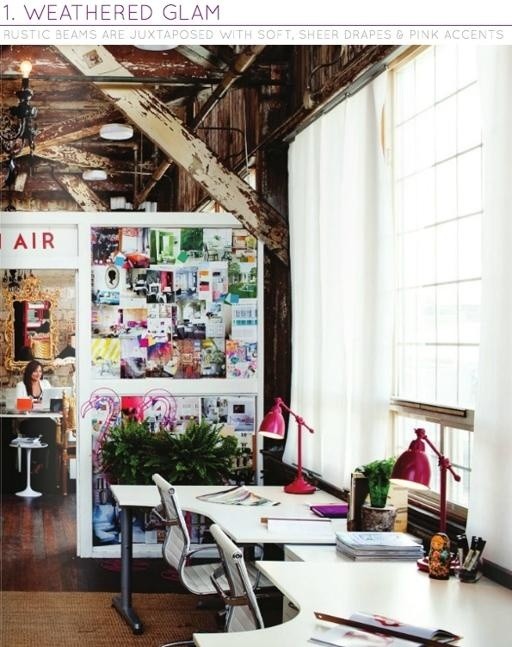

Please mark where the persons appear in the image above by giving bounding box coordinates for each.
[16,360,53,474]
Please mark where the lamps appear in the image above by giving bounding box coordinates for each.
[258,397,316,493]
[0,60,38,212]
[387,427,461,575]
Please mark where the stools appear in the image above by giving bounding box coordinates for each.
[10,442,49,497]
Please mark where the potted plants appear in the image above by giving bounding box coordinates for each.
[354,455,396,532]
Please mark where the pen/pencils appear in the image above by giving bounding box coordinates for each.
[456,534,486,580]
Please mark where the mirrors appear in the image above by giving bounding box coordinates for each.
[3,276,62,372]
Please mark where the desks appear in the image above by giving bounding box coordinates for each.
[1,411,63,488]
[108,484,512,645]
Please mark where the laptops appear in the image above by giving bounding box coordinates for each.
[34,386,72,411]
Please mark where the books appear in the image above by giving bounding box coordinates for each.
[309,505,348,518]
[311,608,461,647]
[334,530,426,562]
